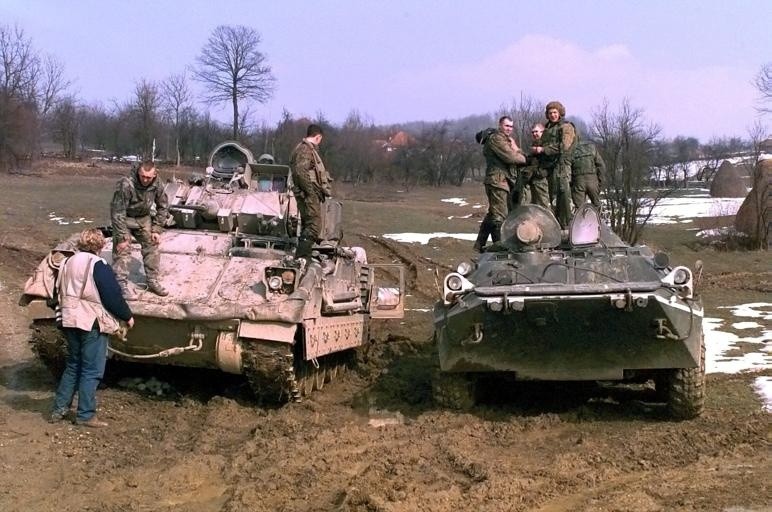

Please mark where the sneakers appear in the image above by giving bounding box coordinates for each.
[47,408,76,423]
[472,242,484,253]
[147,279,169,295]
[121,287,138,300]
[298,258,326,269]
[74,414,108,428]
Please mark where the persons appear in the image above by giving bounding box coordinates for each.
[569,140,607,212]
[110,160,169,300]
[529,101,577,226]
[472,116,527,252]
[52,227,135,428]
[288,123,332,268]
[522,123,550,210]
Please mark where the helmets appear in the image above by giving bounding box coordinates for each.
[476,128,498,144]
[546,102,565,119]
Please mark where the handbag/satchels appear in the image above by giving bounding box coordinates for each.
[312,161,334,196]
[55,304,62,330]
[540,161,556,177]
